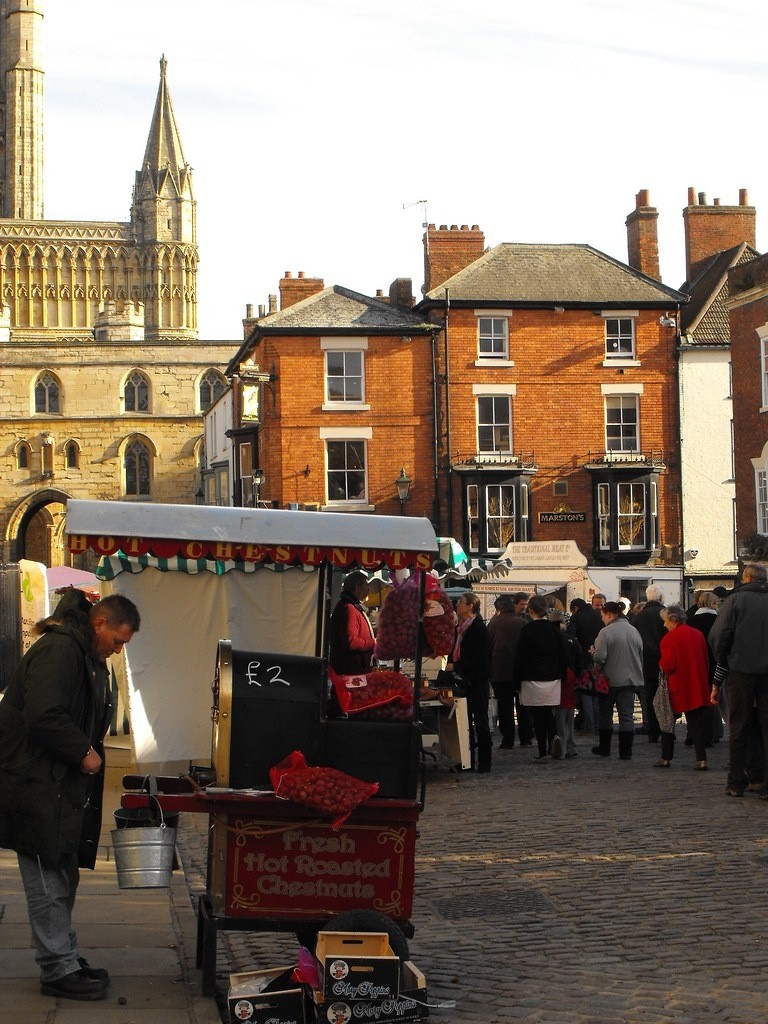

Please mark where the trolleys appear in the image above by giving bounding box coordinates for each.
[63,497,439,968]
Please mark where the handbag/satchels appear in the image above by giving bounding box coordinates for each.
[518,678,562,707]
[653,669,674,734]
[433,667,465,689]
[572,649,609,698]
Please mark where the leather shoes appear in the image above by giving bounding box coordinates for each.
[43,955,110,1001]
[693,760,706,771]
[653,758,670,767]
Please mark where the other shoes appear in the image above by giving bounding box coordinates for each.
[685,736,692,747]
[520,739,532,748]
[533,748,578,763]
[550,735,562,758]
[635,723,650,735]
[704,735,719,749]
[724,782,768,800]
[499,744,513,750]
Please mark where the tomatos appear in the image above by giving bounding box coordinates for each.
[278,587,456,814]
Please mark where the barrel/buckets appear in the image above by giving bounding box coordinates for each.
[113,773,182,871]
[110,795,176,889]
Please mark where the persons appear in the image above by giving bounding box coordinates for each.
[446,563,768,797]
[327,569,380,678]
[0,591,141,1000]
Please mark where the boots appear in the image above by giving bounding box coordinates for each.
[470,732,492,772]
[618,729,633,760]
[590,729,611,757]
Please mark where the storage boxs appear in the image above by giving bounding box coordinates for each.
[317,931,400,1001]
[229,966,307,1024]
[313,961,430,1024]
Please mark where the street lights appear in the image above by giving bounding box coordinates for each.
[394,469,412,516]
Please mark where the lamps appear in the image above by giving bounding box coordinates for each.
[395,468,411,503]
